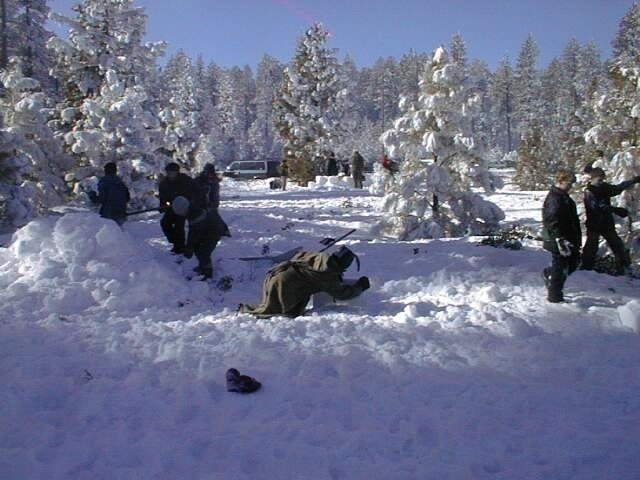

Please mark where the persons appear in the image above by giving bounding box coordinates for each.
[541,168,582,302]
[237,246,370,318]
[324,151,364,189]
[88,162,232,283]
[580,167,639,271]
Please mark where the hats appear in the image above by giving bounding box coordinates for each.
[166,163,179,171]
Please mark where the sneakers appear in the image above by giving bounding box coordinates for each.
[226,368,261,393]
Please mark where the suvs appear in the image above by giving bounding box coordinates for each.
[213,158,284,182]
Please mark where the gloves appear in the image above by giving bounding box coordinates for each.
[356,275,369,291]
[556,237,575,257]
